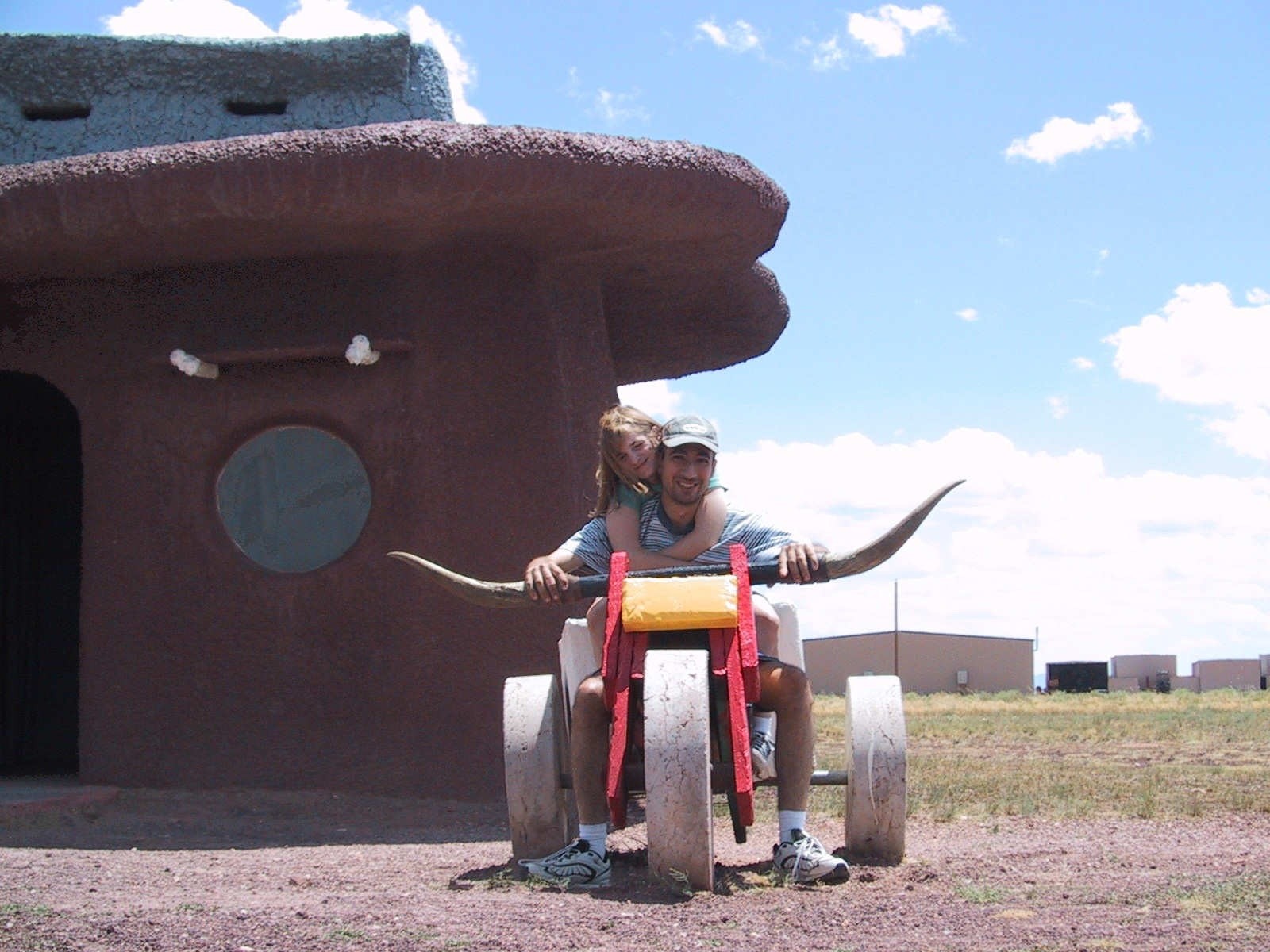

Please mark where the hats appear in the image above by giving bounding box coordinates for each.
[655,413,719,455]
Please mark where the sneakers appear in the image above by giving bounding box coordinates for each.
[516,838,613,889]
[749,729,777,766]
[772,829,851,884]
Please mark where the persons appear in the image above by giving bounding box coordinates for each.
[588,406,780,779]
[508,413,851,888]
